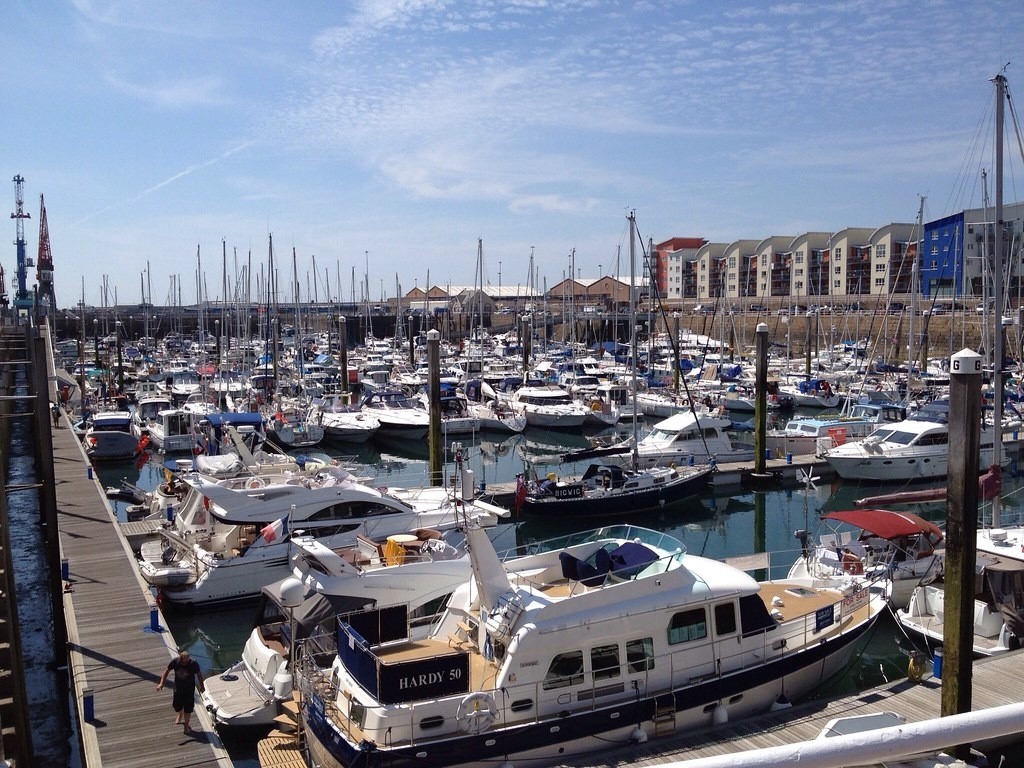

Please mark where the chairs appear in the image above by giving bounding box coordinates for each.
[560,541,660,589]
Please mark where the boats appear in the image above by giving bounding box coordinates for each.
[894,524,1024,655]
[199,530,473,727]
[139,411,515,605]
[282,526,916,768]
[787,508,947,608]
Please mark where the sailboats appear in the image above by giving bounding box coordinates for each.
[54,233,1024,525]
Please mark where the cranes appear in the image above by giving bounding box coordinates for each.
[8,172,36,309]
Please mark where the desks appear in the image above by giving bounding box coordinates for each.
[386,533,418,550]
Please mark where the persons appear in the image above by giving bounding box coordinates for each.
[51,403,62,428]
[156,651,206,733]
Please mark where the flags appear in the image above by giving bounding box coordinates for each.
[260,514,290,544]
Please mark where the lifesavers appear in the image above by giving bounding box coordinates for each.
[841,553,862,576]
[456,692,497,734]
[591,403,602,412]
[245,477,265,493]
[809,389,816,396]
[682,399,689,406]
[875,385,882,391]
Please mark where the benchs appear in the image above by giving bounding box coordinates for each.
[356,529,443,568]
